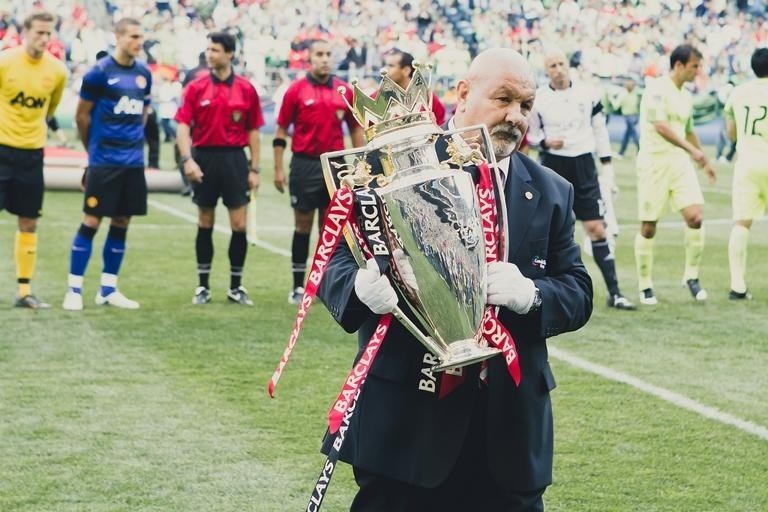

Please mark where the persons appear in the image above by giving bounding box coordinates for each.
[0,0,768,312]
[314,45,594,511]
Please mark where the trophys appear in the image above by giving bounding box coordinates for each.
[319,58,509,373]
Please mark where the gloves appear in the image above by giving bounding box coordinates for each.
[353,256,399,317]
[487,260,536,314]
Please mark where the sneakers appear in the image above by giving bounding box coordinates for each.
[681,276,707,301]
[288,286,305,306]
[13,292,51,309]
[94,288,140,311]
[728,287,754,301]
[226,284,255,307]
[191,285,212,305]
[62,288,83,311]
[639,286,658,306]
[603,292,638,309]
